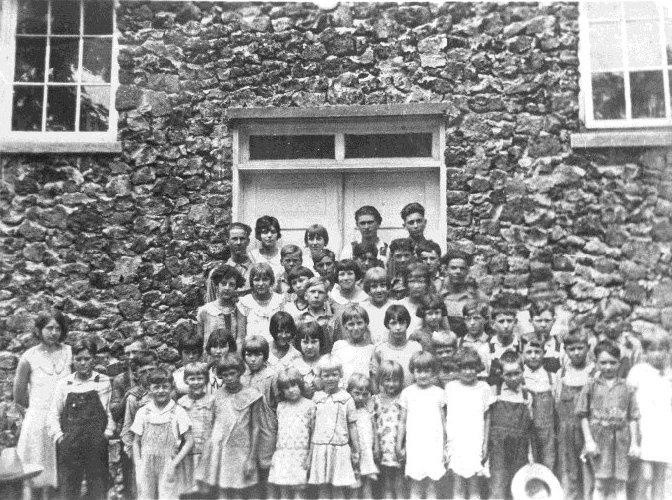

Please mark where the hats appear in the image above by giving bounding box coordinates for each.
[510,462,566,500]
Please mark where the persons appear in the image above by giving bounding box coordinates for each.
[14,309,216,500]
[479,268,671,500]
[199,202,495,500]
